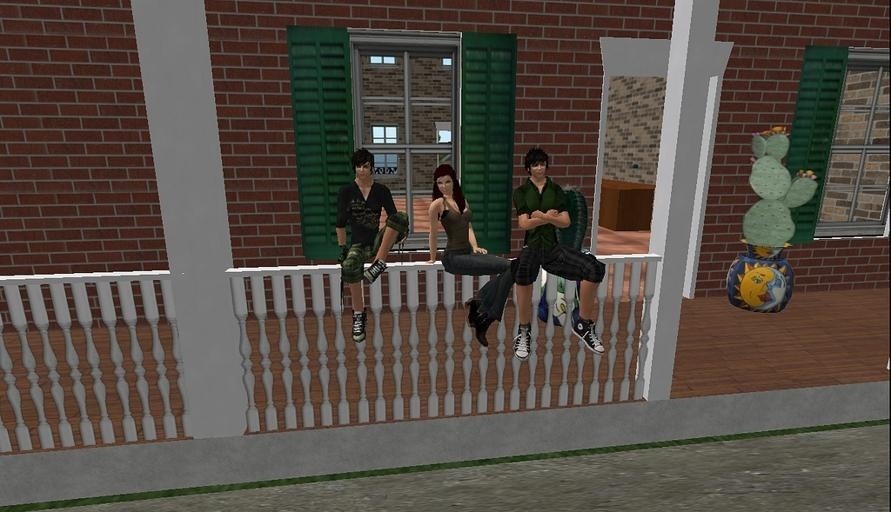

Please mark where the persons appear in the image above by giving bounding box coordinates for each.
[511,146,607,360]
[427,163,518,347]
[334,146,411,347]
